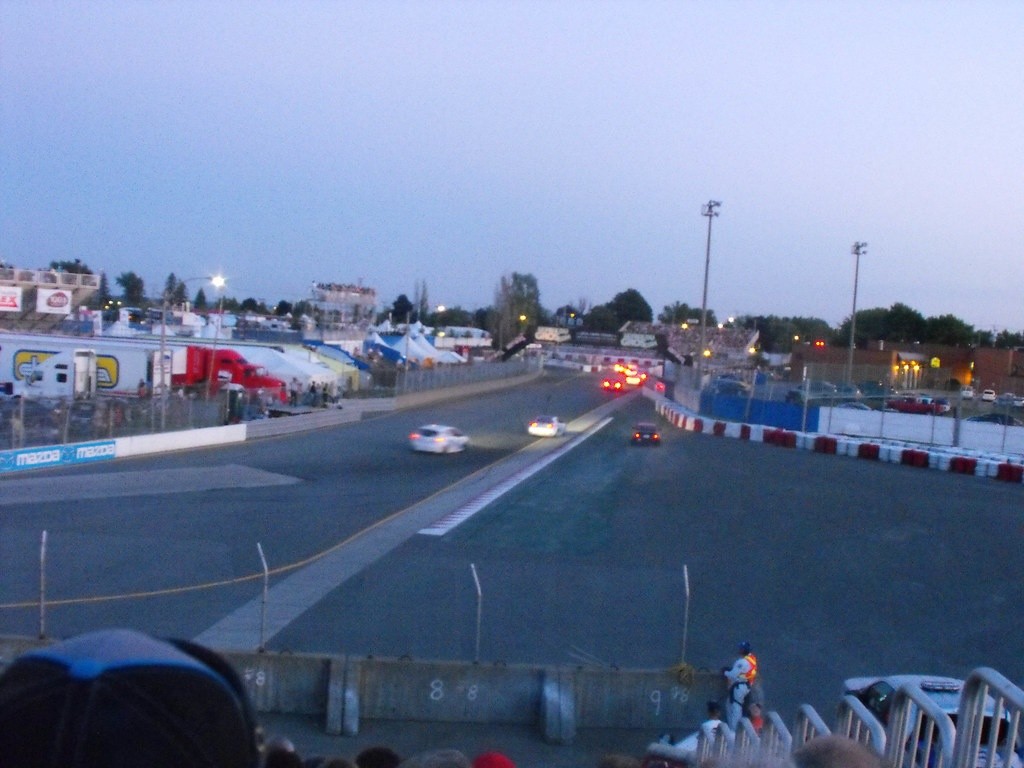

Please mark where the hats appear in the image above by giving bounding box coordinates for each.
[473,753,517,768]
[0,631,257,768]
[738,642,751,650]
[706,700,720,713]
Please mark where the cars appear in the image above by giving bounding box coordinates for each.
[798,378,1024,426]
[630,422,662,445]
[410,424,471,453]
[527,415,567,438]
[600,362,648,392]
[844,674,1024,768]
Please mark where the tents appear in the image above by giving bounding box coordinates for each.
[361,332,467,367]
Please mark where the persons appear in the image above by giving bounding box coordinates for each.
[700,639,763,737]
[290,377,329,409]
[0,629,892,767]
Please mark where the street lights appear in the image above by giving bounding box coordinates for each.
[698,199,721,360]
[160,275,224,399]
[846,240,868,381]
